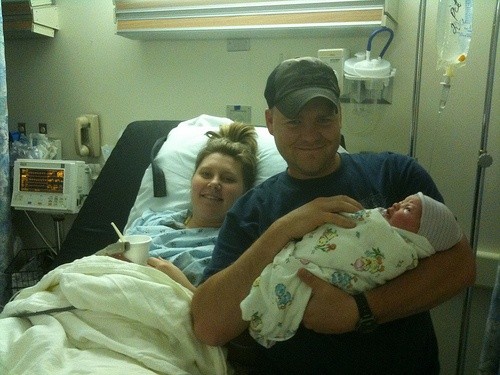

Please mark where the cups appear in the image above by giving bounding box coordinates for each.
[118,235,152,266]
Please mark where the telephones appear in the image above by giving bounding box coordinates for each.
[74,113,100,158]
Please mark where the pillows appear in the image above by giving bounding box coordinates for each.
[126,115,350,233]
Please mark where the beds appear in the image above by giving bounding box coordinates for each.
[57,120,304,375]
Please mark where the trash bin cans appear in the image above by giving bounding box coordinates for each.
[5,247,58,296]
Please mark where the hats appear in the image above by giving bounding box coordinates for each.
[415,192,465,253]
[263,56,341,120]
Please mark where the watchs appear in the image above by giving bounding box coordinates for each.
[353,293,377,334]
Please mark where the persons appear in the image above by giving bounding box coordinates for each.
[191,55,476,375]
[240,192,464,349]
[119,121,258,293]
